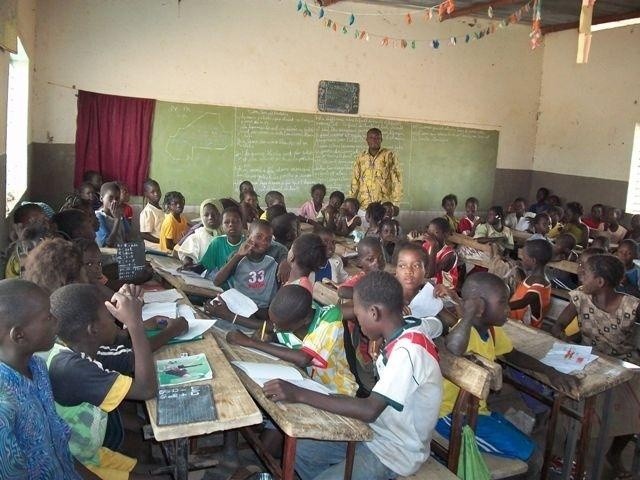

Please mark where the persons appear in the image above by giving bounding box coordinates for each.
[347,127,403,229]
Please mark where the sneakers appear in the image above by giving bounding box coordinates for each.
[569,459,590,480]
[548,455,564,475]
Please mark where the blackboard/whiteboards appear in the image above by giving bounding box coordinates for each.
[156,384,218,426]
[118,241,147,282]
[318,80,359,114]
[149,99,500,214]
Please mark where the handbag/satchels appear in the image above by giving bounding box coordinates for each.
[116,239,154,287]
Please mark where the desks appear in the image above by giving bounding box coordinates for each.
[496,320,639,480]
[136,252,374,479]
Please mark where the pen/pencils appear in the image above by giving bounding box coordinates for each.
[176,303,179,318]
[261,320,266,342]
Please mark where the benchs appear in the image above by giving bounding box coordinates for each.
[544,259,580,300]
[512,231,533,259]
[450,232,504,270]
[314,280,530,479]
